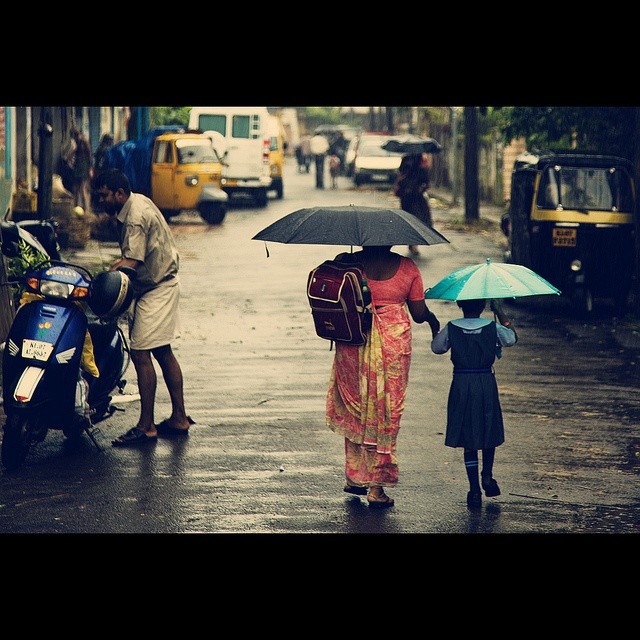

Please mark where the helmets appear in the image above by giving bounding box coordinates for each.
[87,268,134,320]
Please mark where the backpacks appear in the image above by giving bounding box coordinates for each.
[306,252,374,352]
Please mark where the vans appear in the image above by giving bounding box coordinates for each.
[354,136,402,183]
[188,107,272,206]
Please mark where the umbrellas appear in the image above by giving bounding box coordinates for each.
[379,132,446,168]
[426,258,563,324]
[251,201,451,258]
[314,125,339,133]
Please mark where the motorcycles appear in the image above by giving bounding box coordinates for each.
[2,220,130,467]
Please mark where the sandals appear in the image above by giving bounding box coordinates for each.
[343,485,368,496]
[368,493,395,508]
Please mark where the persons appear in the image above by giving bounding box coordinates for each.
[292,133,311,174]
[328,130,350,189]
[69,127,95,212]
[430,294,517,507]
[310,129,329,190]
[59,135,77,191]
[324,230,439,509]
[329,151,342,186]
[398,153,434,259]
[95,166,190,446]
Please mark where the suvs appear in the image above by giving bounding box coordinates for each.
[268,117,283,198]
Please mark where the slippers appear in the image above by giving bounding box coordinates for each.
[155,421,189,437]
[110,427,157,447]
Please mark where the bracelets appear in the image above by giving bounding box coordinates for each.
[502,321,511,327]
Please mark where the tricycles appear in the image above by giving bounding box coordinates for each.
[102,133,228,224]
[511,150,637,315]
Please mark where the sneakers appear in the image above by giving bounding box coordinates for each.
[467,492,482,508]
[482,477,501,497]
